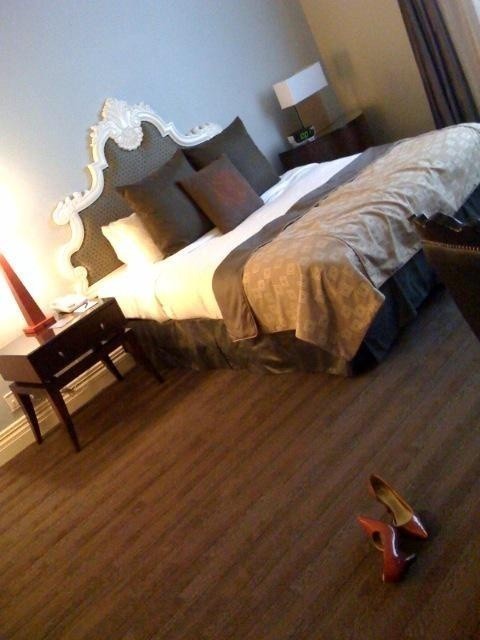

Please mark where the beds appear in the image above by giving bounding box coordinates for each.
[47,91,480,384]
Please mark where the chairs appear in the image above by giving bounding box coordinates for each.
[404,203,480,341]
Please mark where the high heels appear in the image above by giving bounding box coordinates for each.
[357,515,419,583]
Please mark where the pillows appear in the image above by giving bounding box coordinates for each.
[179,113,285,196]
[111,146,217,261]
[173,150,267,237]
[94,207,166,273]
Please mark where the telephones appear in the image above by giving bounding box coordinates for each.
[52,295,88,314]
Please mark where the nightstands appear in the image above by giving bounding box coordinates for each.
[0,292,165,455]
[274,106,376,174]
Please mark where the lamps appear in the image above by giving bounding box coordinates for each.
[268,57,338,144]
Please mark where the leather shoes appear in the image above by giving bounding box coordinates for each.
[368,474,429,539]
